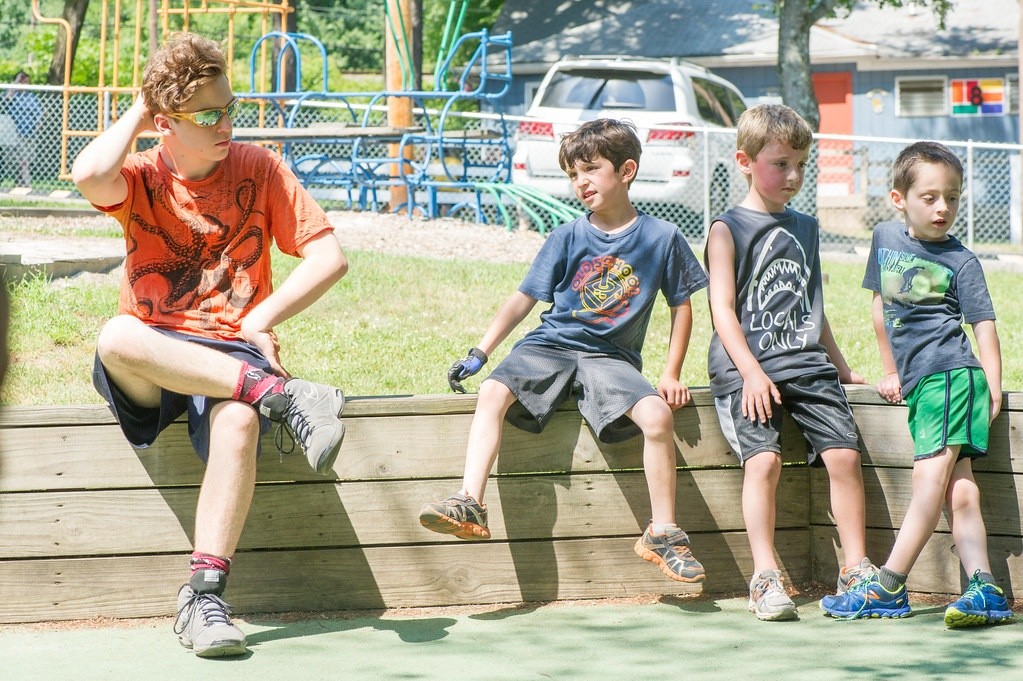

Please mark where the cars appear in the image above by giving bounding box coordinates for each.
[509,54,751,233]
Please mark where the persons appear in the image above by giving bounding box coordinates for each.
[818,143,1015,627]
[74,32,348,657]
[704,104,880,620]
[8,72,40,185]
[421,119,708,583]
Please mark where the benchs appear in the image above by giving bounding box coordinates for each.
[0,384,813,626]
[810,384,1023,600]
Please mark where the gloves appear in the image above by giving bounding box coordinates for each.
[447,347,489,394]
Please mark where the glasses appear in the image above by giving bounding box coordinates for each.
[166,95,241,128]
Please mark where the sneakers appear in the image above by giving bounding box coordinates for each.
[835,556,881,596]
[748,572,799,621]
[818,571,913,621]
[943,568,1015,629]
[418,489,491,541]
[173,568,247,656]
[634,518,707,583]
[258,376,346,477]
